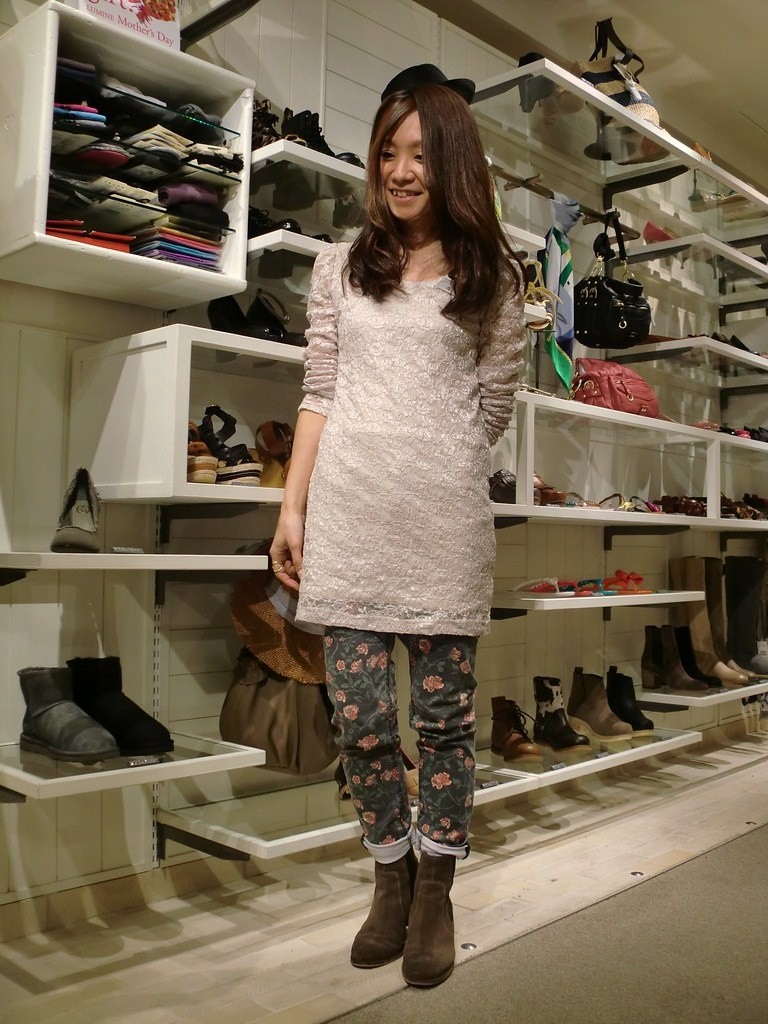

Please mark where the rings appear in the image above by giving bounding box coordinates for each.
[272,561,284,573]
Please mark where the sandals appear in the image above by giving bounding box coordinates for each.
[688,181,768,221]
[196,405,264,487]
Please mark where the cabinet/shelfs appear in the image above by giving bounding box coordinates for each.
[0,0,768,863]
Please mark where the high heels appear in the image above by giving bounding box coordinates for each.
[187,404,217,484]
[50,466,107,553]
[248,204,332,279]
[642,220,768,291]
[246,289,308,347]
[252,98,371,228]
[206,296,280,367]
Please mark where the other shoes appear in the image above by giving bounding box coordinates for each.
[538,84,585,124]
[517,53,555,113]
[490,468,768,530]
[686,330,768,378]
[685,421,768,442]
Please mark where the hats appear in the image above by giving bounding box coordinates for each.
[381,63,476,110]
[227,538,328,685]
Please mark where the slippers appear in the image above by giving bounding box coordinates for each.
[507,568,654,599]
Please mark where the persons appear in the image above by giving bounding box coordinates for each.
[270,62,524,988]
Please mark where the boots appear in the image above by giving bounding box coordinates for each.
[669,555,761,685]
[14,666,119,764]
[723,555,768,678]
[66,656,177,754]
[490,623,723,764]
[349,844,456,987]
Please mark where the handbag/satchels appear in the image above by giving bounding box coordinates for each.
[569,18,674,162]
[219,650,342,776]
[571,356,660,418]
[573,211,653,350]
[248,421,295,488]
